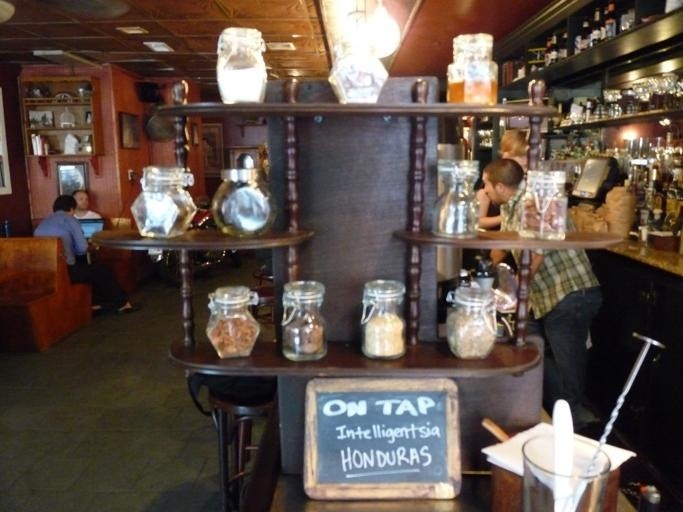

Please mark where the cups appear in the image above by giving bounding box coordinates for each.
[520,434,611,512]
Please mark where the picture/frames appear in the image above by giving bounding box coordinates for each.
[28,110,55,130]
[197,121,225,177]
[55,161,89,204]
[0,88,14,197]
[119,112,141,149]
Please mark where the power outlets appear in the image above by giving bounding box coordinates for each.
[128,169,135,182]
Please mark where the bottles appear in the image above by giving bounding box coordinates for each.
[205,284,261,360]
[278,279,331,363]
[518,167,569,244]
[209,168,282,239]
[215,26,269,105]
[430,159,481,242]
[443,283,501,361]
[130,163,199,240]
[329,24,390,105]
[446,31,499,107]
[358,279,408,362]
[543,0,682,67]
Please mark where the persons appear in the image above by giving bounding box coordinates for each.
[479,158,604,418]
[473,128,531,231]
[71,189,106,242]
[33,194,143,317]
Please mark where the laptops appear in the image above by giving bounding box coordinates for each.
[77,218,106,241]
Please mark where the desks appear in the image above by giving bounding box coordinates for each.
[239,397,640,512]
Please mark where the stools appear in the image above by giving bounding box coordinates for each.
[207,380,278,510]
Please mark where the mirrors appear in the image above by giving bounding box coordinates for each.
[227,144,262,169]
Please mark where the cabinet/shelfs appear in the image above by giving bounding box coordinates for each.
[164,76,544,379]
[464,2,681,245]
[591,250,683,478]
[19,76,98,160]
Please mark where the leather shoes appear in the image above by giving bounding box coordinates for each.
[94,305,113,314]
[120,303,140,313]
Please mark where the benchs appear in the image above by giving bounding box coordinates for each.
[0,235,92,353]
[27,217,133,294]
